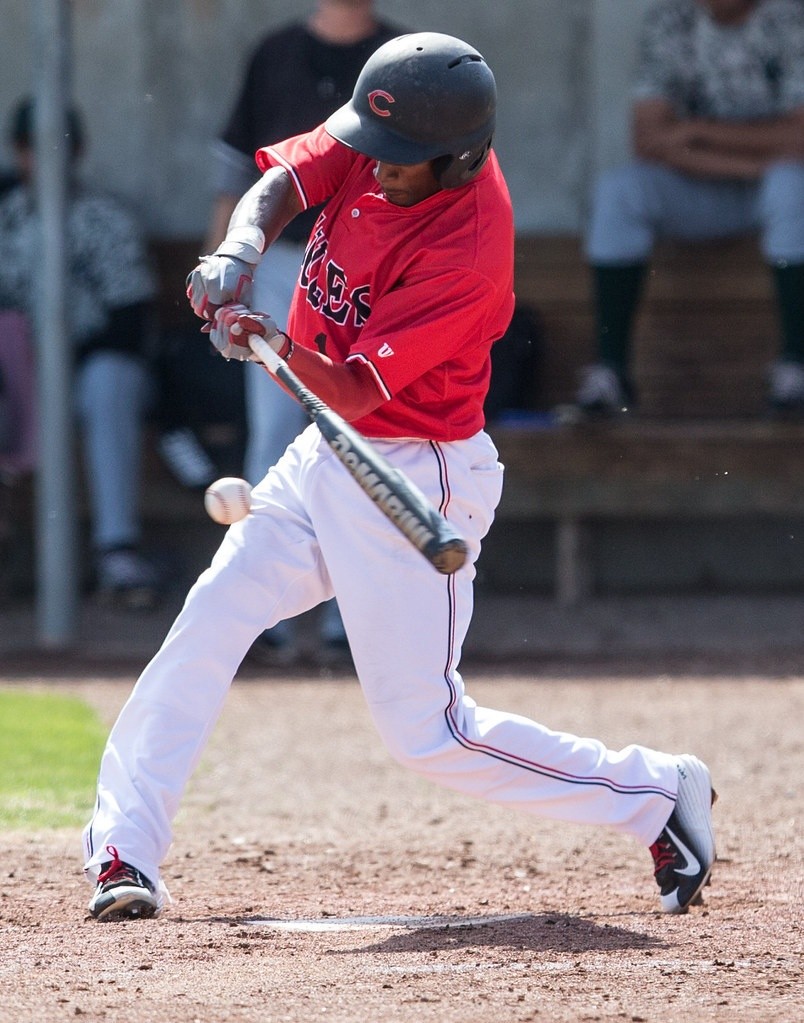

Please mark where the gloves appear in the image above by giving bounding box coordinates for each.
[186,241,261,333]
[211,303,285,362]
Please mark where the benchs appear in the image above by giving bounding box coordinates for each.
[0,411,804,608]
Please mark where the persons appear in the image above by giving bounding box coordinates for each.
[0,94,152,600]
[210,0,406,654]
[83,33,716,926]
[576,0,803,404]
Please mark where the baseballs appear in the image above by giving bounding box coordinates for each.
[204,476,252,525]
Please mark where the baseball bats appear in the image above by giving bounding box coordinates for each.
[248,333,470,577]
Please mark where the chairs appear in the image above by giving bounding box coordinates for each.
[206,194,783,419]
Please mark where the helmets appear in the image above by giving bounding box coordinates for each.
[324,31,497,188]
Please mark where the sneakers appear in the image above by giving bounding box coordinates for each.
[650,754,717,914]
[89,865,158,919]
